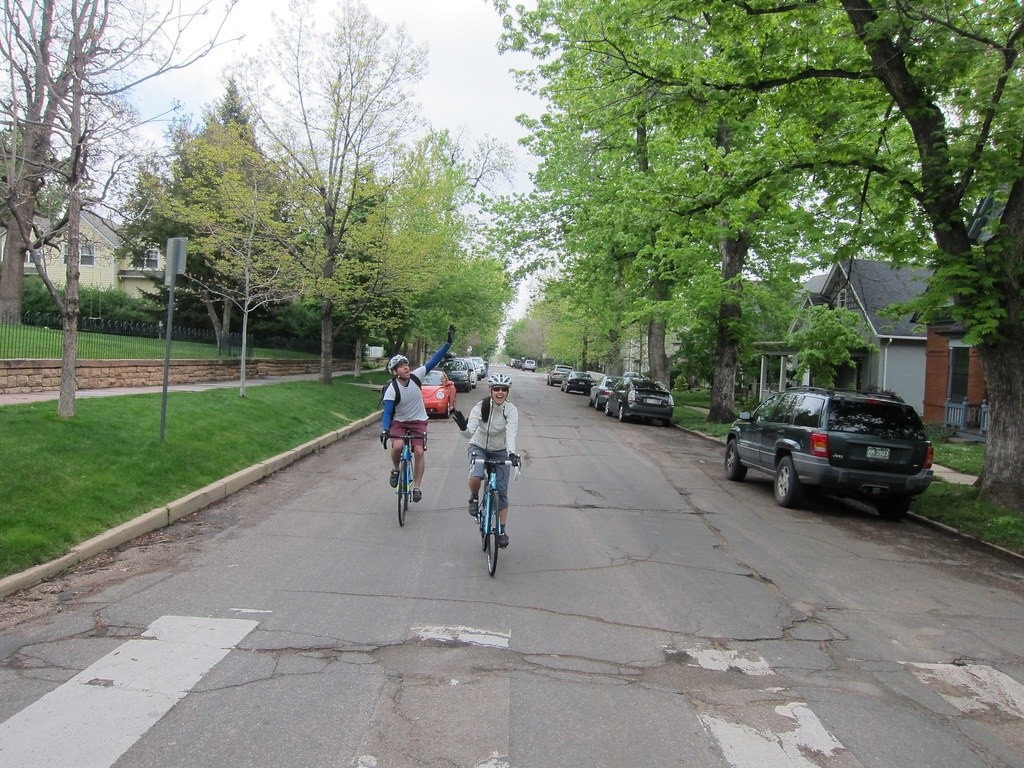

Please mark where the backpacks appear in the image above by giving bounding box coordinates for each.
[377,373,422,411]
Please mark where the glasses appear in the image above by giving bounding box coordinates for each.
[492,386,508,392]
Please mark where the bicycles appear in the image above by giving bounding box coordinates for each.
[380,431,427,527]
[468,453,522,576]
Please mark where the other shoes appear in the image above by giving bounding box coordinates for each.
[468,497,479,517]
[389,468,400,488]
[498,533,509,547]
[413,487,422,502]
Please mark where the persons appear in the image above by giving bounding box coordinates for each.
[379,323,457,502]
[159,320,163,339]
[453,373,519,547]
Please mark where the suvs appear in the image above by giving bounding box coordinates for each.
[522,360,537,372]
[546,365,573,386]
[724,386,935,521]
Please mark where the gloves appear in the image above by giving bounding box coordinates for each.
[509,452,519,467]
[380,431,390,443]
[452,409,469,431]
[447,324,457,343]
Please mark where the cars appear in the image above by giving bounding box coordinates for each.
[468,357,486,381]
[561,370,592,396]
[420,371,457,419]
[604,378,674,425]
[589,376,620,409]
[624,372,645,378]
[464,359,479,389]
[440,359,474,392]
[507,359,523,369]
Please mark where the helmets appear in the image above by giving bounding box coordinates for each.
[387,355,410,375]
[488,372,512,388]
